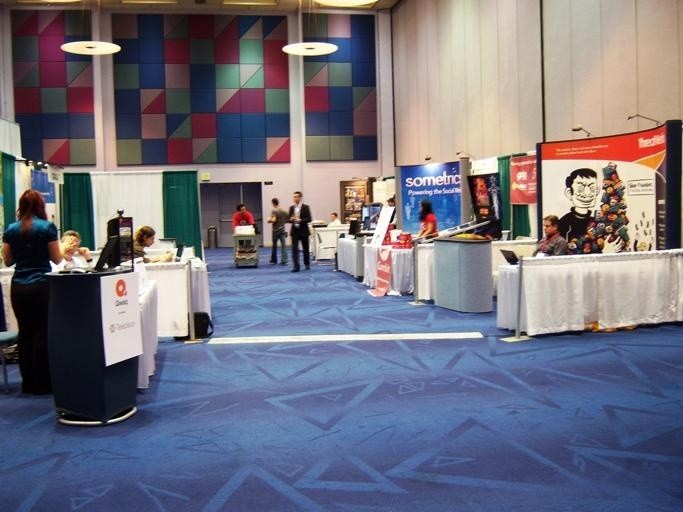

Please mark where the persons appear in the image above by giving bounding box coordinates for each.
[289,192,313,273]
[416,200,438,242]
[50,230,96,274]
[329,213,341,227]
[3,189,63,395]
[232,205,256,246]
[387,199,398,229]
[533,214,569,256]
[267,198,289,265]
[558,168,624,256]
[128,226,174,264]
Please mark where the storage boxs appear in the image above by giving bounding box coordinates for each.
[0,331,19,393]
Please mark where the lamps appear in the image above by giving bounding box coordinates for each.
[456,150,475,160]
[571,124,593,137]
[60,0,122,56]
[281,0,338,56]
[627,113,663,127]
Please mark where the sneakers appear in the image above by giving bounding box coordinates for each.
[279,262,288,266]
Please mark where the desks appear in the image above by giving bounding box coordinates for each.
[496,264,523,330]
[337,238,362,279]
[362,243,415,294]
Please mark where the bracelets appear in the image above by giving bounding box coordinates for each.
[88,257,94,264]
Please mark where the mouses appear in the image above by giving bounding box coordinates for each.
[72,268,85,273]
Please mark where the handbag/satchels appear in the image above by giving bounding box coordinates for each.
[174,312,214,342]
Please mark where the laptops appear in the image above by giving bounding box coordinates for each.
[501,250,520,265]
[176,245,183,258]
[76,235,118,273]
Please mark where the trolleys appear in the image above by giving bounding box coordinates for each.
[232,224,259,269]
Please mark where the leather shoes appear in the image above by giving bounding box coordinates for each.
[305,262,310,270]
[291,268,300,272]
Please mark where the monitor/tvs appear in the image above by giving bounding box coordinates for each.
[349,220,361,239]
[361,205,370,222]
